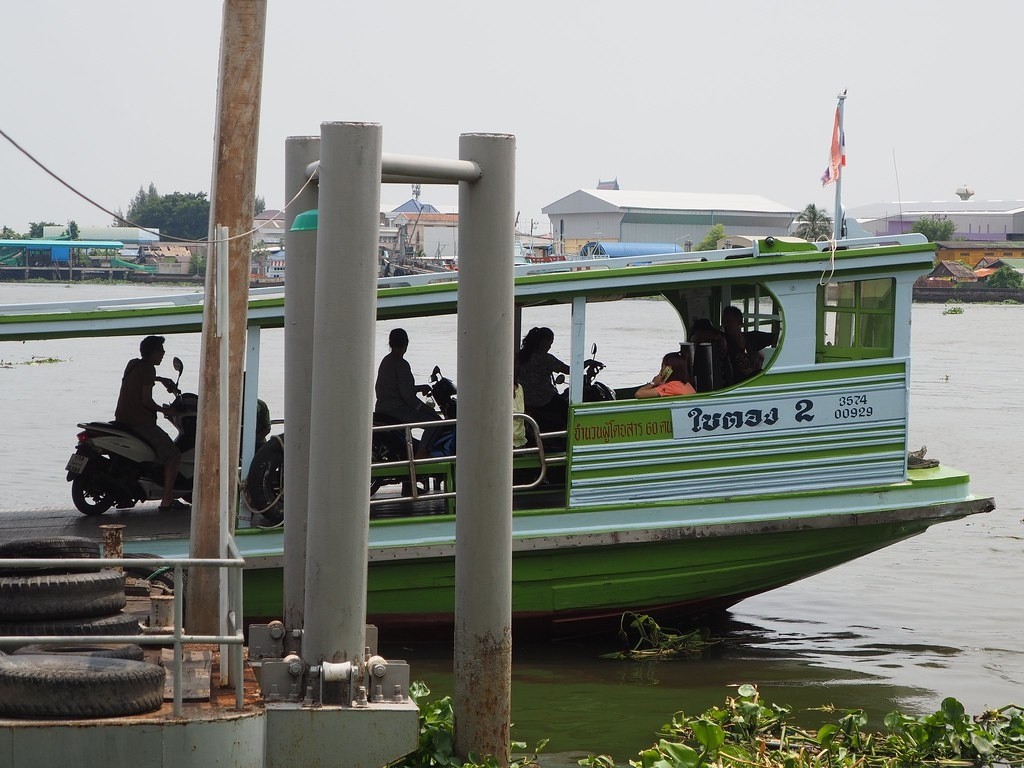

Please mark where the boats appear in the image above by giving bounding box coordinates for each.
[0,229,998,638]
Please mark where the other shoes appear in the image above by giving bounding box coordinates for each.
[401,483,427,496]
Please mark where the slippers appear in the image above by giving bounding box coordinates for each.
[158,500,189,511]
[116,501,135,508]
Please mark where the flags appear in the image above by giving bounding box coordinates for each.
[820,102,847,188]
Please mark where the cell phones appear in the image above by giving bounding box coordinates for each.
[660,366,673,382]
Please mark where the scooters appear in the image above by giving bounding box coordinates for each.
[525,342,618,452]
[65,356,199,517]
[371,365,458,496]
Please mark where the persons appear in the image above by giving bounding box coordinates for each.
[635,352,698,399]
[255,401,271,454]
[114,337,191,515]
[693,306,780,388]
[376,329,441,458]
[513,328,597,458]
[384,266,391,277]
